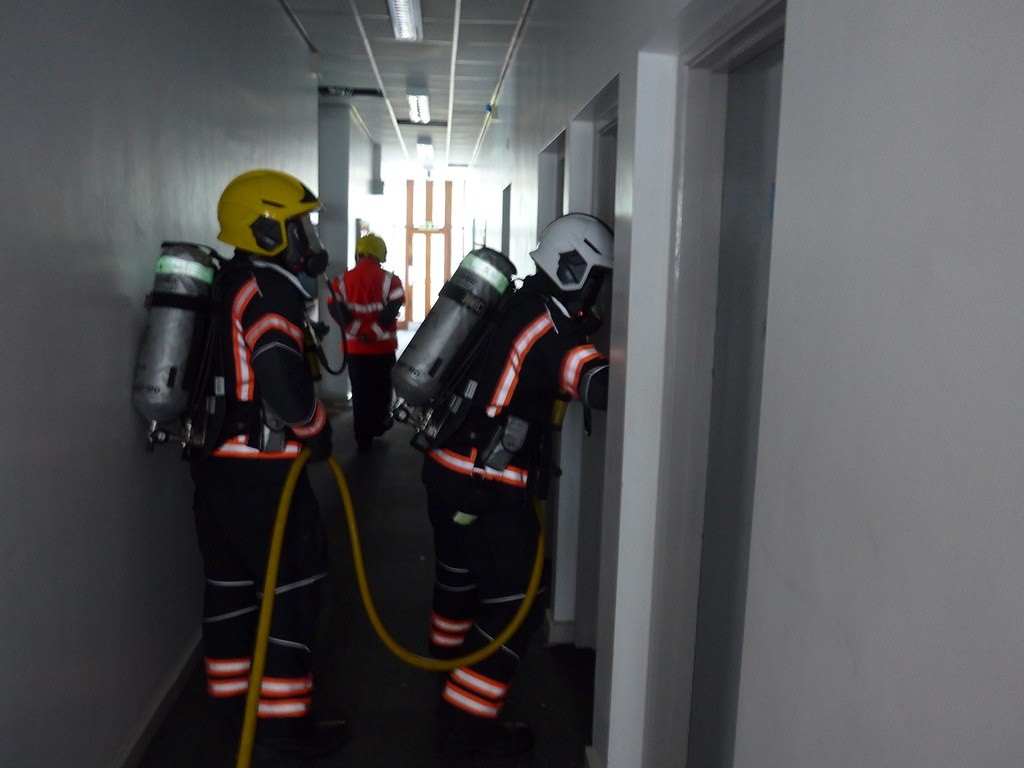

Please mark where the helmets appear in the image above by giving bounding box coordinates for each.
[530,212,612,291]
[355,235,387,263]
[217,171,320,255]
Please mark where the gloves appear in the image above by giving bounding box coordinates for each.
[299,420,333,464]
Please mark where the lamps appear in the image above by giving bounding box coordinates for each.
[388,0,423,42]
[406,89,430,125]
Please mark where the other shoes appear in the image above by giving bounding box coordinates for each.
[254,719,349,761]
[374,416,396,437]
[435,717,532,761]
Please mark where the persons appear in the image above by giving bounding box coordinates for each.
[423,211,614,760]
[197,170,352,761]
[327,233,405,445]
